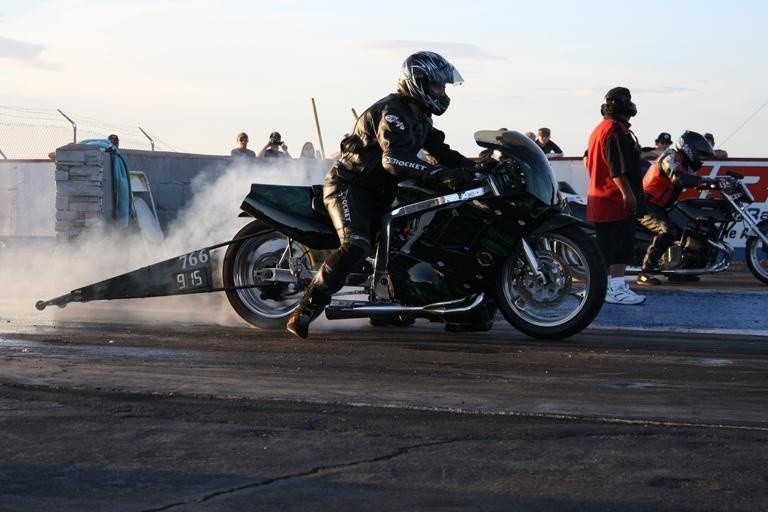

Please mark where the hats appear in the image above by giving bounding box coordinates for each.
[604,87,632,105]
[538,127,551,138]
[657,132,672,145]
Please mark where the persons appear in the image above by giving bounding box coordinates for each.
[640,132,673,161]
[583,87,647,306]
[230,132,256,157]
[107,134,120,148]
[633,130,726,286]
[535,128,563,159]
[703,133,728,159]
[287,50,500,340]
[299,141,315,159]
[257,131,292,158]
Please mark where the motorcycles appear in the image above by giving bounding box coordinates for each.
[221,129,609,341]
[554,167,767,283]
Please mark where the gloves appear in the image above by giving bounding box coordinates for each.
[715,175,738,194]
[425,163,474,193]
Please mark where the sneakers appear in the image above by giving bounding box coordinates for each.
[286,299,317,341]
[605,283,647,305]
[636,271,662,286]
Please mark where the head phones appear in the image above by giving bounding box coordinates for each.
[600,86,637,117]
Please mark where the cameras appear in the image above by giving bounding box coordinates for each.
[273,141,282,145]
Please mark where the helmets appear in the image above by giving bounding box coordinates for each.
[675,130,713,173]
[397,49,465,116]
